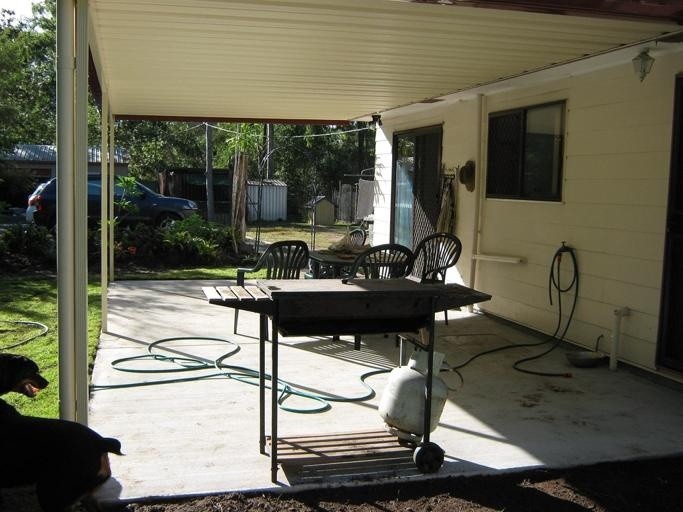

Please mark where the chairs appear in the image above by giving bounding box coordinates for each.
[330,242,417,354]
[229,239,311,338]
[306,228,368,279]
[404,231,463,327]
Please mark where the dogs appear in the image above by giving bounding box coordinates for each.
[0,353,126,511]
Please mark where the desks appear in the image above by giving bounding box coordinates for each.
[200,278,495,482]
[303,246,404,277]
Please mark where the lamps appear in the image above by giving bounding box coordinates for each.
[630,47,655,84]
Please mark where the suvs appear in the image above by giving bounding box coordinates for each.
[25,182,101,222]
[33,172,200,232]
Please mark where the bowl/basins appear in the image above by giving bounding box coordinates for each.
[563,350,604,368]
